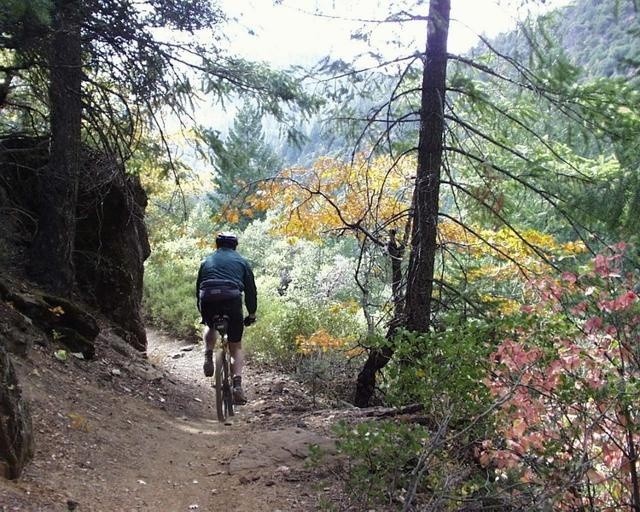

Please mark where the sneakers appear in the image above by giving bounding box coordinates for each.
[203,359,214,377]
[233,386,248,403]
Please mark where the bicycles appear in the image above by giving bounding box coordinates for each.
[198,315,257,421]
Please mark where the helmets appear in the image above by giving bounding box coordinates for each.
[215,231,238,248]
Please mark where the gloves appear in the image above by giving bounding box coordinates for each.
[244,314,256,327]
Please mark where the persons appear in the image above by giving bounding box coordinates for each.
[194,231,258,404]
